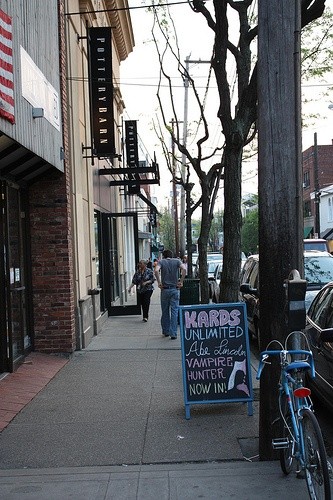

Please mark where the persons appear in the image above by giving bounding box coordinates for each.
[154,250,186,339]
[146,257,158,270]
[129,260,154,322]
[181,256,188,279]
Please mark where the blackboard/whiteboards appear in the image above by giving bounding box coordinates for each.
[179,302,255,404]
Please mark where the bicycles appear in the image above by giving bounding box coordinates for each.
[255,331,331,500]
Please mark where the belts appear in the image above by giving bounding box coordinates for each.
[163,286,179,289]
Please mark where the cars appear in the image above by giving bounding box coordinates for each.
[208,263,245,303]
[192,253,199,264]
[195,254,224,286]
[302,239,330,252]
[238,251,333,348]
[305,282,333,408]
